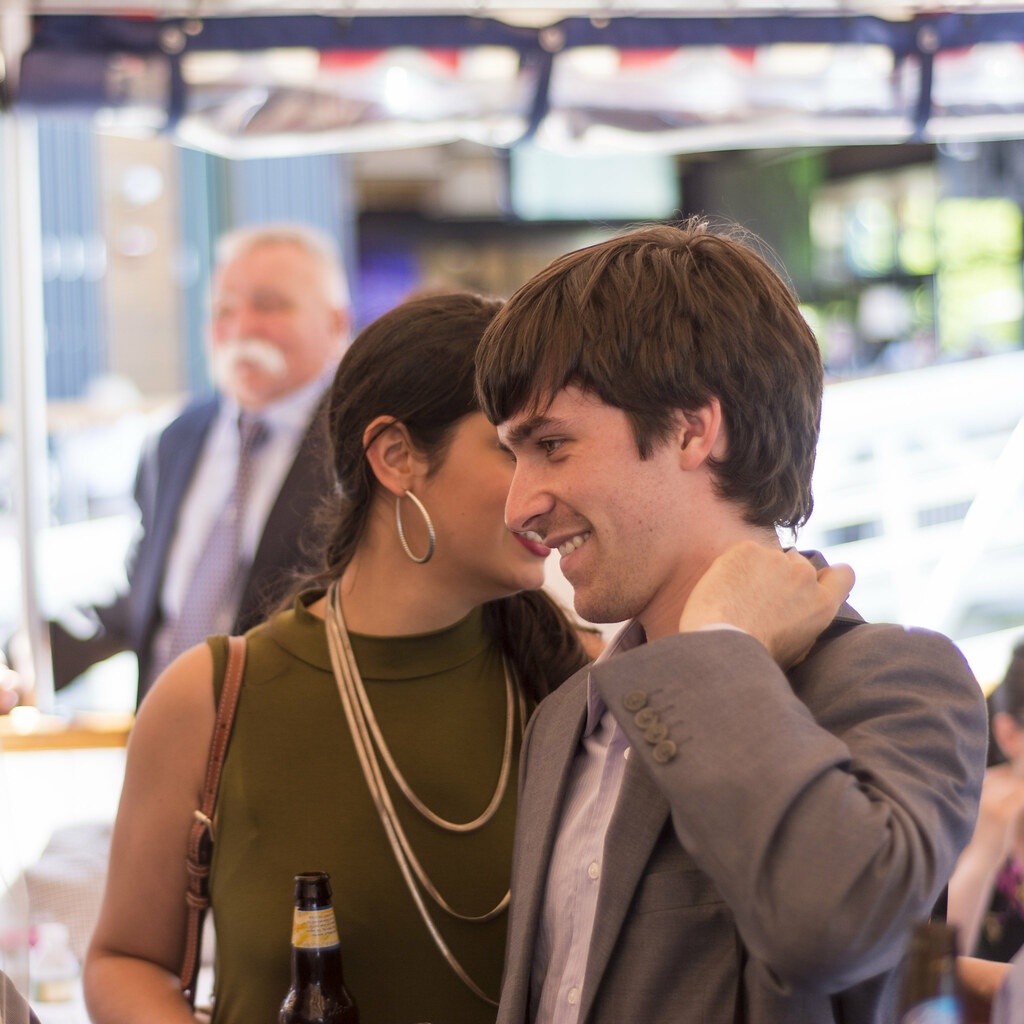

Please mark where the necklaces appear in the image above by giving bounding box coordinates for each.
[324,582,530,1007]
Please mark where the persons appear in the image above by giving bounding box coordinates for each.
[474,222,988,1024]
[0,229,351,719]
[947,639,1023,1024]
[83,293,610,1024]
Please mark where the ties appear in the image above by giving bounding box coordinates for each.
[166,413,274,670]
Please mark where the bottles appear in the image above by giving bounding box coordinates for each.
[892,920,971,1024]
[275,872,361,1024]
